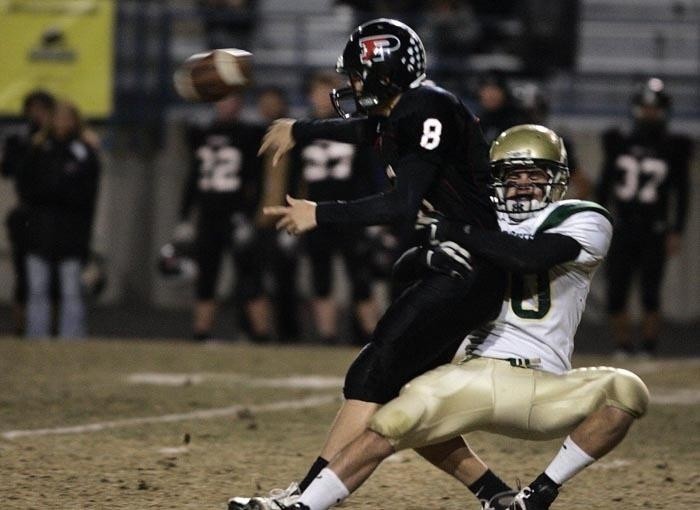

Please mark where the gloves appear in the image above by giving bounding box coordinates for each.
[412,210,476,285]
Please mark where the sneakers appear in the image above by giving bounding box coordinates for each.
[228,482,311,510]
[480,478,559,510]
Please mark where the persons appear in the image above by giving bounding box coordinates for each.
[245,72,588,343]
[591,78,691,361]
[228,16,519,510]
[251,125,649,510]
[0,89,104,339]
[174,95,276,342]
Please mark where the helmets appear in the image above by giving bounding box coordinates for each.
[335,17,426,101]
[628,77,673,116]
[489,123,571,201]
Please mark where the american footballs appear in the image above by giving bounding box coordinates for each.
[162,49,254,103]
